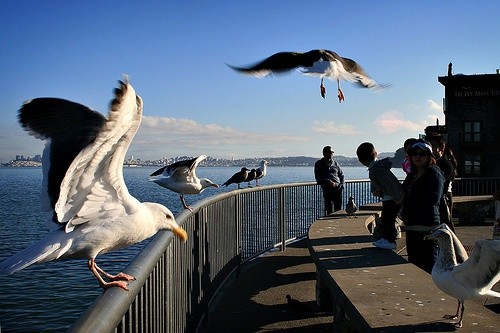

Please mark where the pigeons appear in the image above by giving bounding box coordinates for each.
[345,197,358,219]
[221,160,268,189]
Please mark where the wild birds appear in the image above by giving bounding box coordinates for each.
[148,154,219,215]
[0,73,188,292]
[423,222,499,328]
[223,48,393,104]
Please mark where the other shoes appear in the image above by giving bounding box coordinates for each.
[373,238,396,249]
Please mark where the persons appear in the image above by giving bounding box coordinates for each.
[401,132,457,274]
[314,146,345,216]
[357,142,405,249]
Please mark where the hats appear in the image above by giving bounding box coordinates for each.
[412,141,434,152]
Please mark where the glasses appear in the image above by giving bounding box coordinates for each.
[412,150,427,156]
